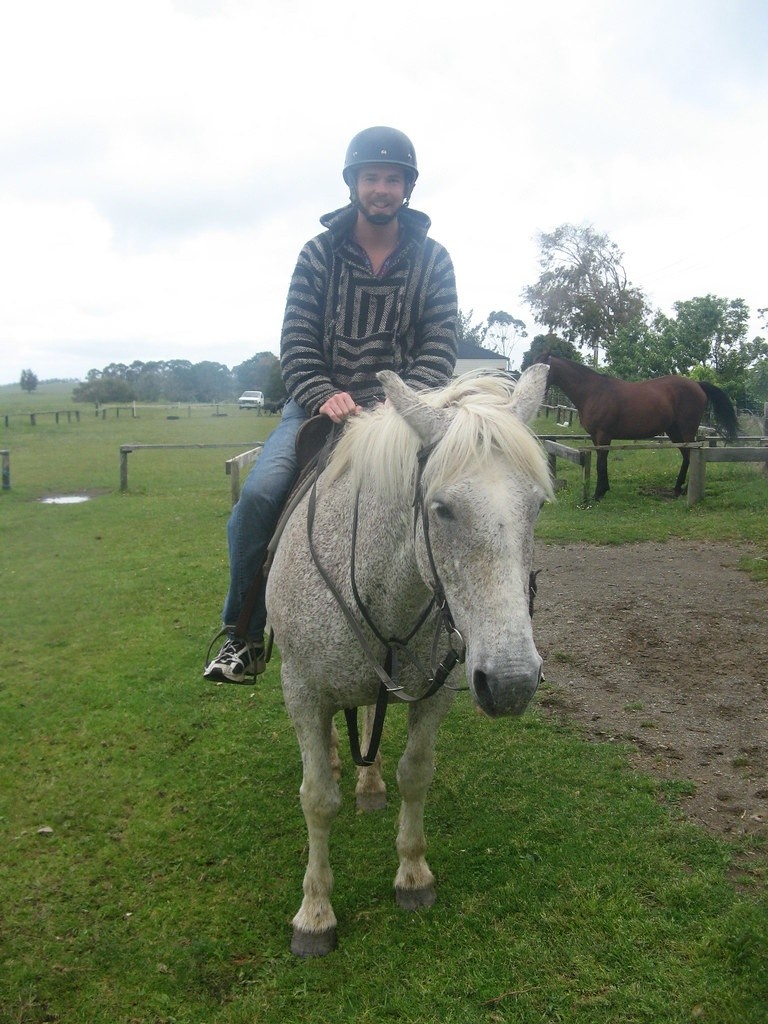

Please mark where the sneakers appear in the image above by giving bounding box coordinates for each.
[203,639,266,683]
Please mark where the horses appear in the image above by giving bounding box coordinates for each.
[265,364,557,957]
[533,350,747,503]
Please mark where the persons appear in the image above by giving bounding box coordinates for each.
[204,129,457,684]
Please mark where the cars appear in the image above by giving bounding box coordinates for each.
[238,390,265,408]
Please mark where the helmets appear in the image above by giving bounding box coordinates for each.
[343,126,418,175]
